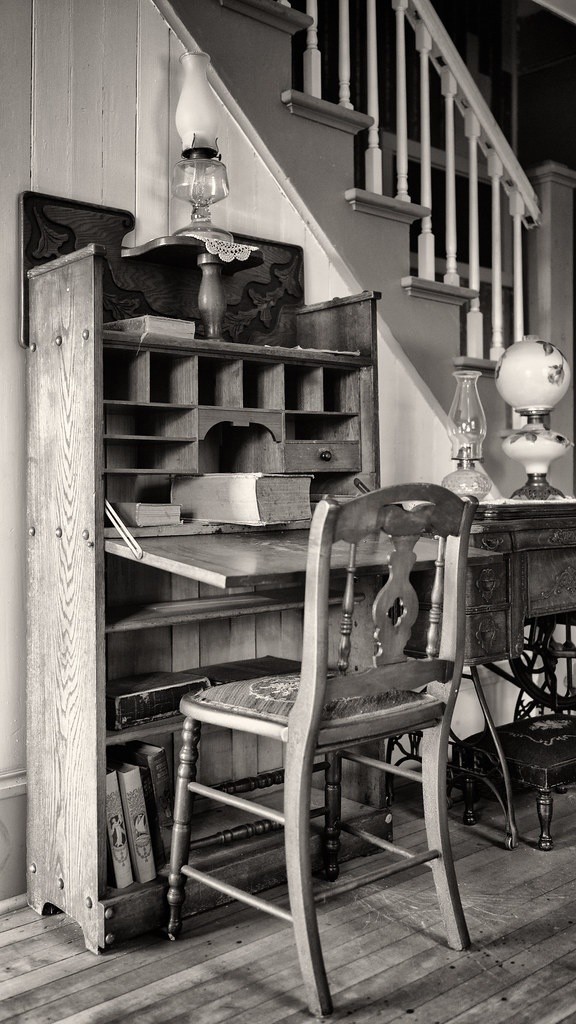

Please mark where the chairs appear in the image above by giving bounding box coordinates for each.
[166,482,479,1022]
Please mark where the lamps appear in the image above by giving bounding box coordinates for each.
[171,51,234,244]
[492,334,574,501]
[445,371,492,502]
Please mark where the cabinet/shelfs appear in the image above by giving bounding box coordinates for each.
[26,244,383,958]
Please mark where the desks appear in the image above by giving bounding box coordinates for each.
[393,501,576,851]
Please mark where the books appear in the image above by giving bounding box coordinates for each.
[103,315,196,339]
[106,740,174,889]
[107,671,211,731]
[109,501,183,527]
[170,472,315,526]
